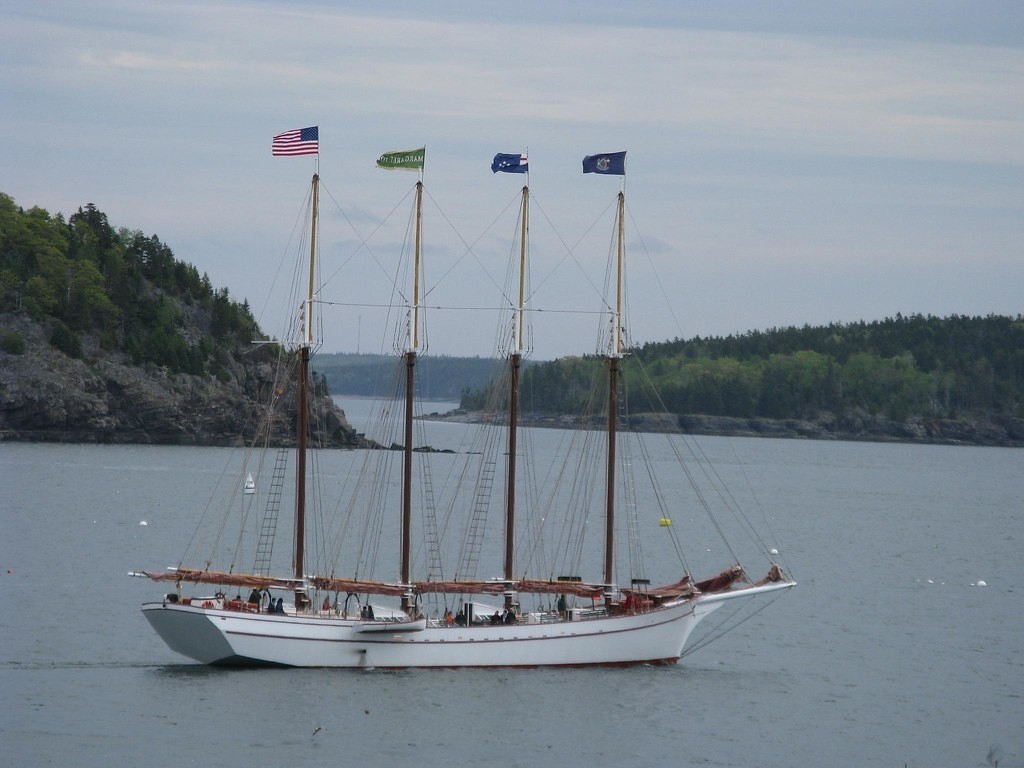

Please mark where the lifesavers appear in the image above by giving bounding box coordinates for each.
[202,601,215,608]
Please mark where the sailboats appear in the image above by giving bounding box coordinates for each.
[126,126,798,672]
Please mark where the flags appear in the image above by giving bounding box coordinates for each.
[375,148,425,172]
[272,126,318,156]
[583,151,627,175]
[491,152,529,174]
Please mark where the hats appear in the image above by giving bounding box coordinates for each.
[162,594,168,600]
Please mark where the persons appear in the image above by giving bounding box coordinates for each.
[268,598,287,615]
[446,611,454,627]
[558,594,567,619]
[455,610,466,627]
[232,596,246,610]
[361,605,374,620]
[163,594,179,604]
[491,609,516,626]
[249,589,261,612]
